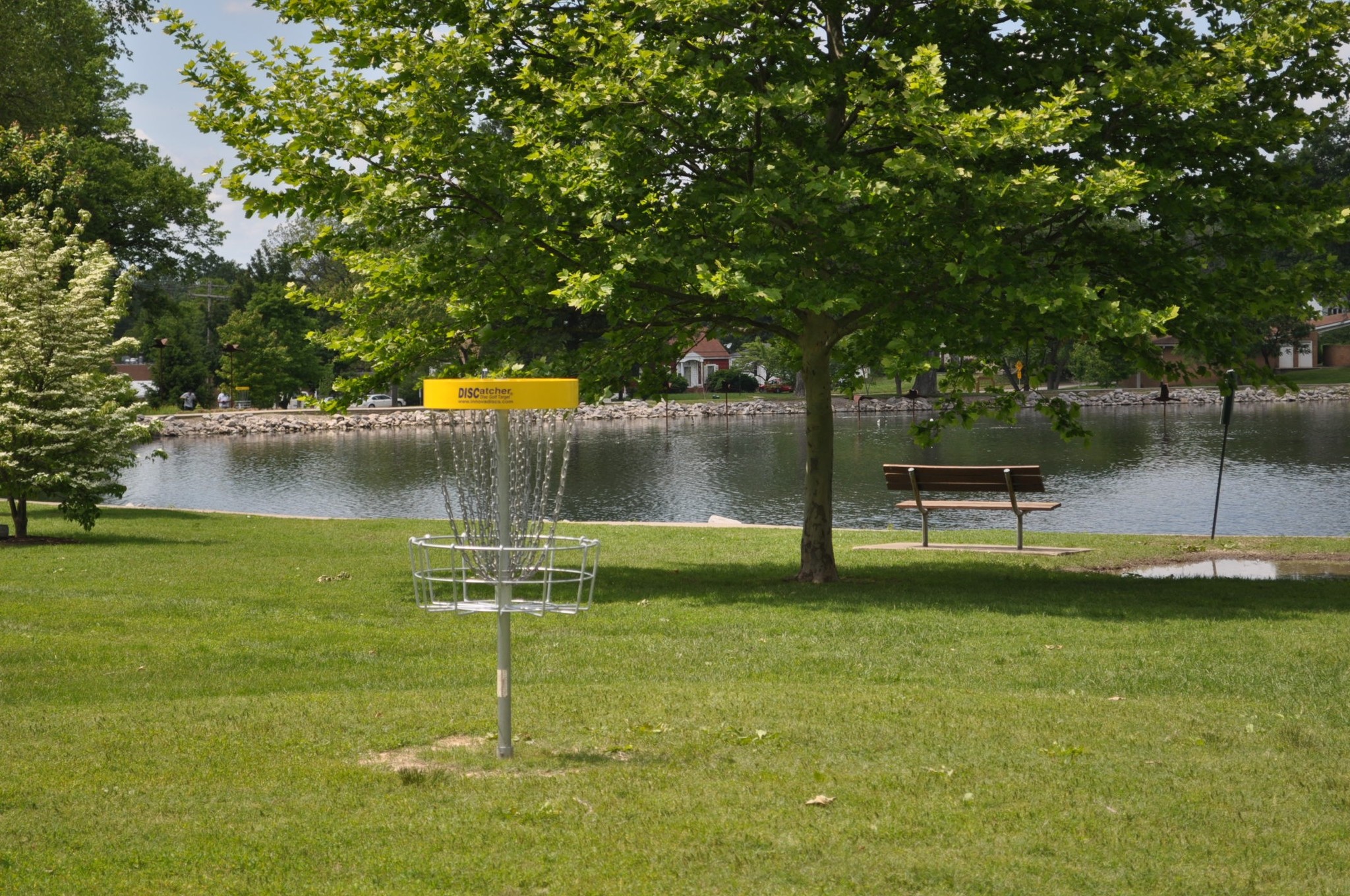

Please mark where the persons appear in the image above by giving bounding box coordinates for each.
[217,392,232,408]
[179,388,197,411]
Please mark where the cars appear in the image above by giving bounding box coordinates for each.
[758,379,793,393]
[287,392,313,409]
[356,393,407,408]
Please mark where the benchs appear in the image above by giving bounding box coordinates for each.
[883,464,1062,550]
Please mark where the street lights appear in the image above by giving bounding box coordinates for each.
[224,342,244,407]
[151,337,175,406]
